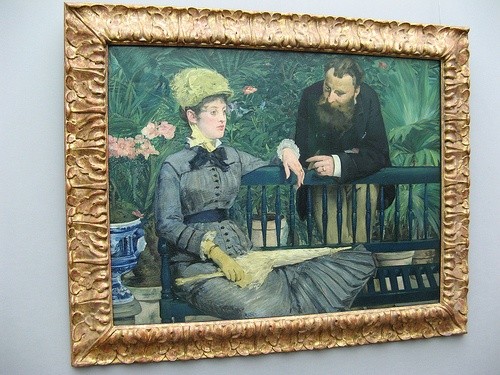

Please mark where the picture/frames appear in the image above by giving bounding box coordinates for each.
[61,1,472,370]
